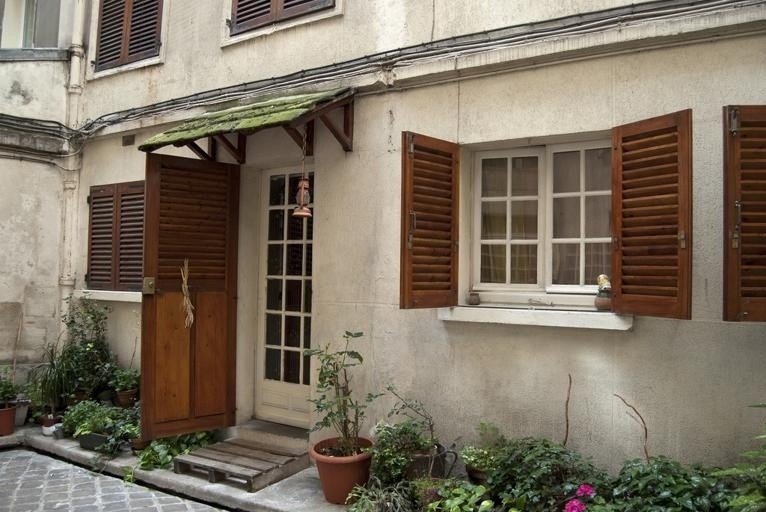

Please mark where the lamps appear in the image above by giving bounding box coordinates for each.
[290,129,315,221]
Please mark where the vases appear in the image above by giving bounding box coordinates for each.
[594,290,612,311]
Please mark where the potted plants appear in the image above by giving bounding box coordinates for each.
[377,382,461,487]
[461,418,504,486]
[1,294,160,455]
[301,329,378,505]
